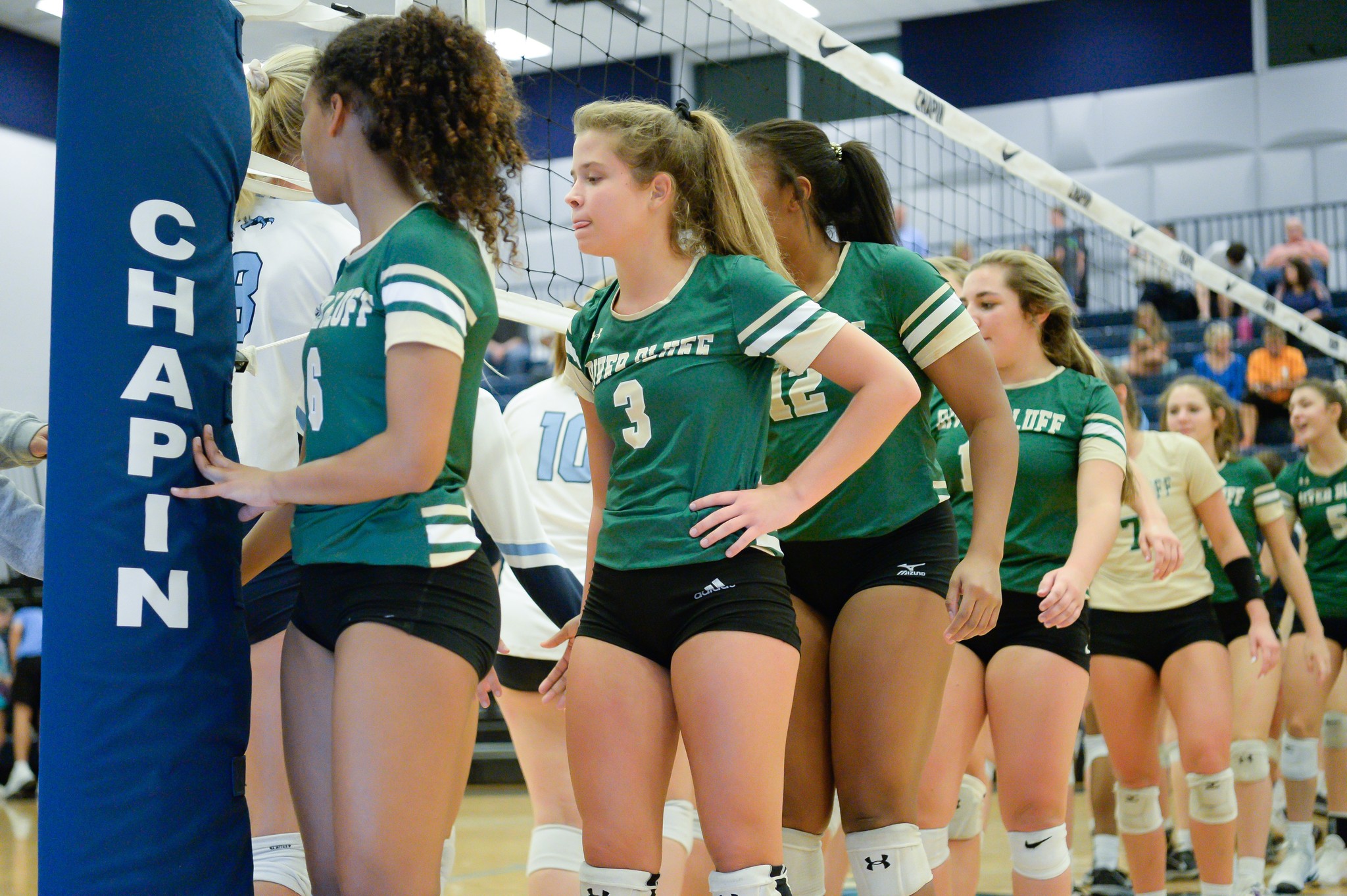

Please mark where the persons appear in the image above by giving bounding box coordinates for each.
[231,50,1346,896]
[0,408,50,798]
[172,6,528,896]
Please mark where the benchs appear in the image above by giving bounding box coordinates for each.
[1072,289,1347,384]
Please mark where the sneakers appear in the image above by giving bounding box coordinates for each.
[1268,845,1319,893]
[1091,868,1133,896]
[1314,834,1347,885]
[0,758,35,799]
[1166,849,1198,881]
